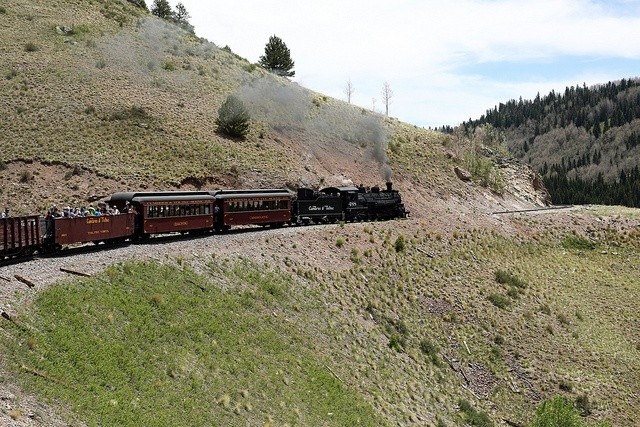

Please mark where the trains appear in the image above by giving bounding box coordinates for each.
[0,182,410,258]
